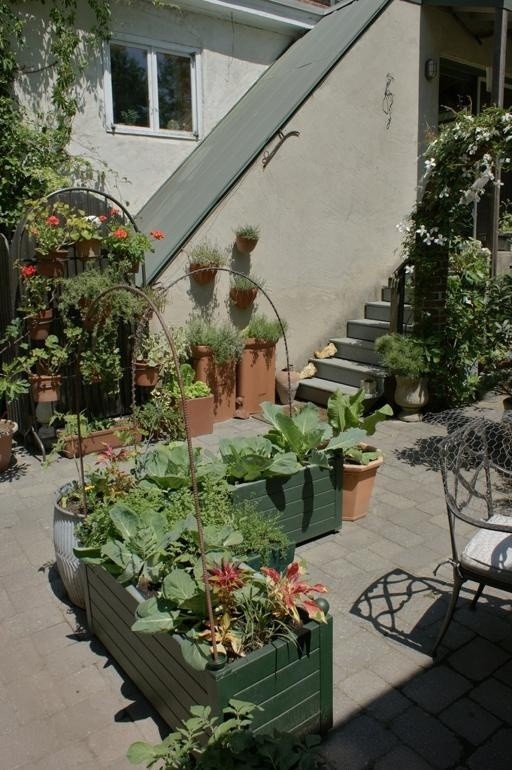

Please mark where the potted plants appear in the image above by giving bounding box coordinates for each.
[187,316,244,422]
[130,331,168,386]
[19,276,56,340]
[328,387,383,521]
[49,410,142,459]
[232,495,296,574]
[19,335,75,402]
[236,313,289,414]
[0,318,44,471]
[235,224,260,252]
[185,247,227,283]
[164,364,214,438]
[376,333,436,422]
[73,444,333,744]
[230,274,262,307]
[216,403,343,545]
[54,442,131,611]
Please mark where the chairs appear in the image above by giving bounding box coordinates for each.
[431,416,512,659]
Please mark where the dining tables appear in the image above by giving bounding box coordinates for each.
[376,333,436,422]
[230,274,262,307]
[185,247,227,283]
[235,224,260,252]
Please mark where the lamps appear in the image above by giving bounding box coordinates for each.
[431,416,512,659]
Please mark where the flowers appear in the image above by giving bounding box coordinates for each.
[395,111,512,259]
[14,208,166,281]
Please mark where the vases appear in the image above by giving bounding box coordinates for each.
[395,111,512,259]
[14,208,166,281]
